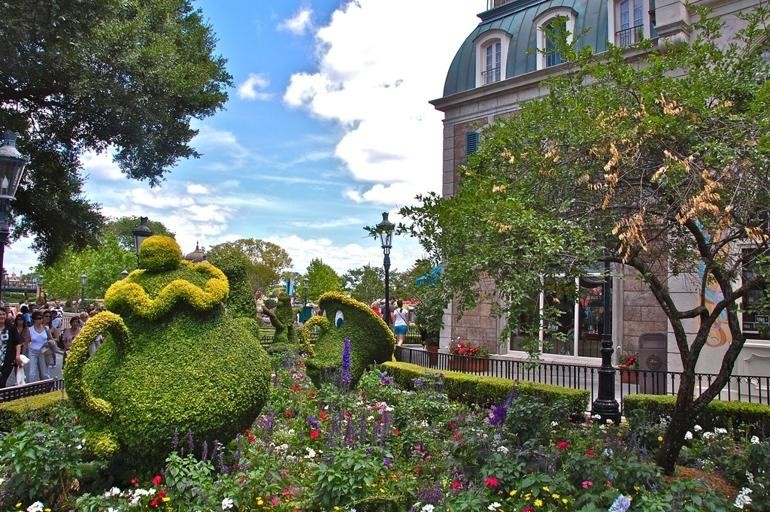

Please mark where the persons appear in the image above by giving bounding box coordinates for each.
[290,295,320,322]
[254,289,270,324]
[0,299,106,388]
[369,296,409,346]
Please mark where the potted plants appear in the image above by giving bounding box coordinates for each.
[413,293,439,365]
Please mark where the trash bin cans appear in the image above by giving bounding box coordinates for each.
[639,333,668,395]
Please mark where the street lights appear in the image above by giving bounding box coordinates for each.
[80,273,87,307]
[132,217,151,264]
[121,269,127,278]
[302,277,308,304]
[376,211,395,326]
[0,130,28,282]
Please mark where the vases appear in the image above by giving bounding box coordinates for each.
[618,365,639,384]
[450,357,487,371]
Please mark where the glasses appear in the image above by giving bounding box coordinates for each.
[34,315,51,319]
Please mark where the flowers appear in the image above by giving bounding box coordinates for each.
[618,351,640,369]
[449,337,492,360]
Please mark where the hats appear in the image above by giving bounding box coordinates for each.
[389,295,396,300]
